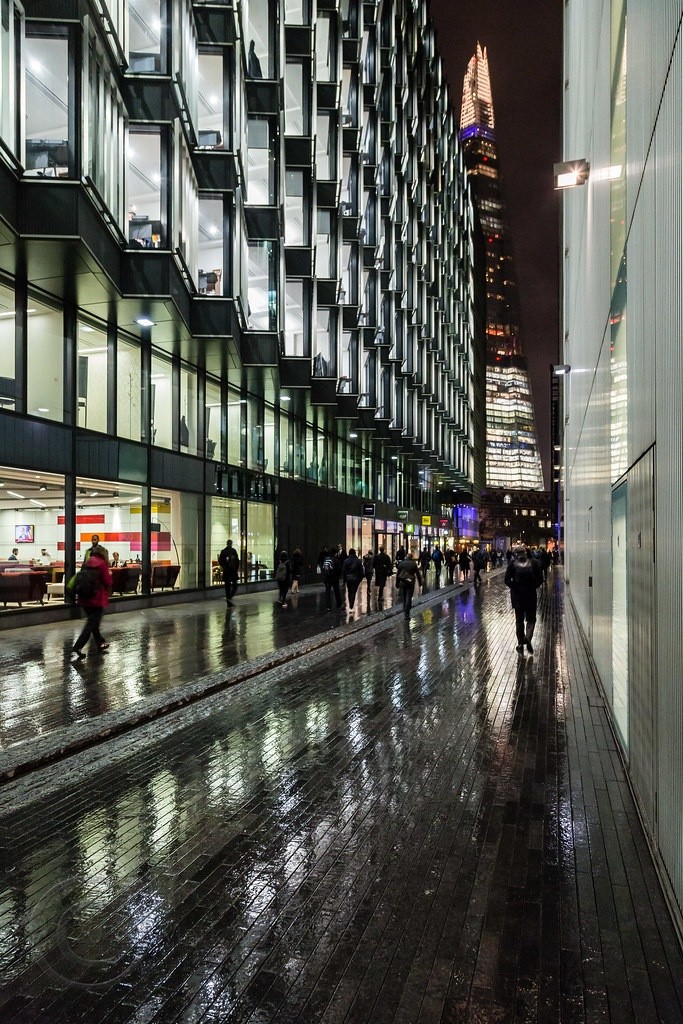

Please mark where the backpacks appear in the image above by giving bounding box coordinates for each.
[74,567,101,602]
[432,550,440,561]
[321,556,335,576]
[275,560,289,581]
[513,557,536,586]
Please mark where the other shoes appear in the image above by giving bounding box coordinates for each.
[516,645,524,654]
[523,636,533,654]
[226,598,235,607]
[277,600,287,607]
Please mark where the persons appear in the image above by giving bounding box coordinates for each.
[81,534,108,564]
[322,548,346,612]
[66,545,112,659]
[396,554,424,620]
[374,547,393,601]
[275,550,291,608]
[504,546,546,655]
[396,545,406,560]
[292,548,302,594]
[7,547,19,561]
[448,543,564,584]
[33,547,52,566]
[109,551,125,569]
[417,546,432,578]
[218,539,240,606]
[433,545,445,580]
[362,549,375,594]
[343,548,363,613]
[334,543,348,586]
[316,545,329,566]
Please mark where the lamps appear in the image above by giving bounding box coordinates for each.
[553,478,560,483]
[550,364,570,377]
[113,492,120,500]
[80,489,86,495]
[554,445,562,451]
[553,465,562,470]
[0,480,5,486]
[14,498,170,513]
[553,158,590,191]
[38,485,47,491]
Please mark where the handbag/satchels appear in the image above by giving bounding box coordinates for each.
[226,554,237,570]
[316,564,321,574]
[346,572,359,583]
[380,559,394,577]
[398,568,416,587]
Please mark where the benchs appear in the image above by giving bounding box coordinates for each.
[0,557,182,608]
[212,560,252,586]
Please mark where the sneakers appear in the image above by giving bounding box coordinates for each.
[72,647,87,658]
[98,643,110,652]
